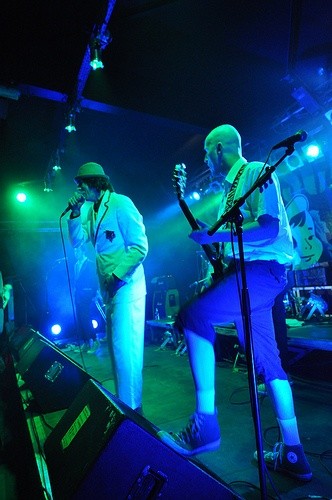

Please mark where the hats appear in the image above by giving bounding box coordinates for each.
[74,162,109,184]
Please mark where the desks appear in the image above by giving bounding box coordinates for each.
[292,286,332,316]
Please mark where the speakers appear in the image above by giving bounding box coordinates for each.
[9,327,246,500]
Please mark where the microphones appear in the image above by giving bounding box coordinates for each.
[61,193,86,216]
[271,129,308,150]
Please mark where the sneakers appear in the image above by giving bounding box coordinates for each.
[255,443,312,480]
[156,407,222,456]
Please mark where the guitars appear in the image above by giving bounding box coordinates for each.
[172,163,228,282]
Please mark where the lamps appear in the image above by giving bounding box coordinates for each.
[300,140,324,163]
[192,179,223,201]
[43,180,53,192]
[89,44,104,69]
[52,156,61,171]
[284,151,304,172]
[64,114,76,132]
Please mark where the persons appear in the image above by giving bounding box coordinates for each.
[156,124,313,482]
[74,246,101,354]
[67,163,148,416]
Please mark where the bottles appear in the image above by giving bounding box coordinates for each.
[155,308,160,321]
